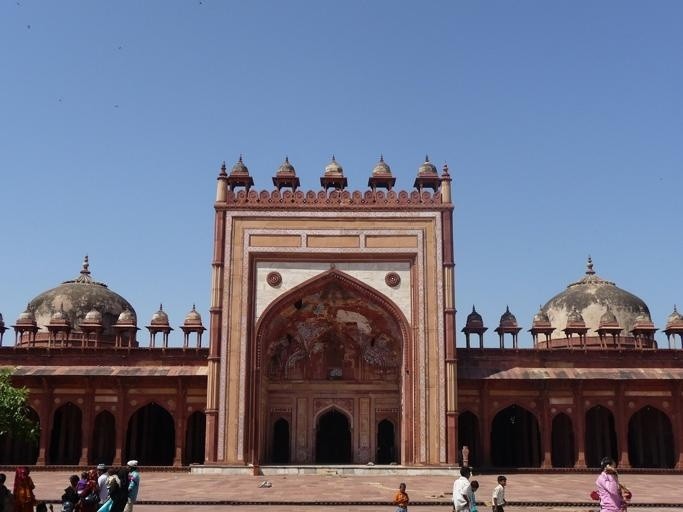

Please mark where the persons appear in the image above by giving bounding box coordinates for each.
[491,476,507,512]
[469,476,479,512]
[596,455,631,512]
[0,457,141,512]
[393,483,408,512]
[452,467,475,512]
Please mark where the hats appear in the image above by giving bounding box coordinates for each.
[127,460,137,468]
[96,464,106,470]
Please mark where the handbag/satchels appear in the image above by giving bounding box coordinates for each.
[82,483,100,507]
